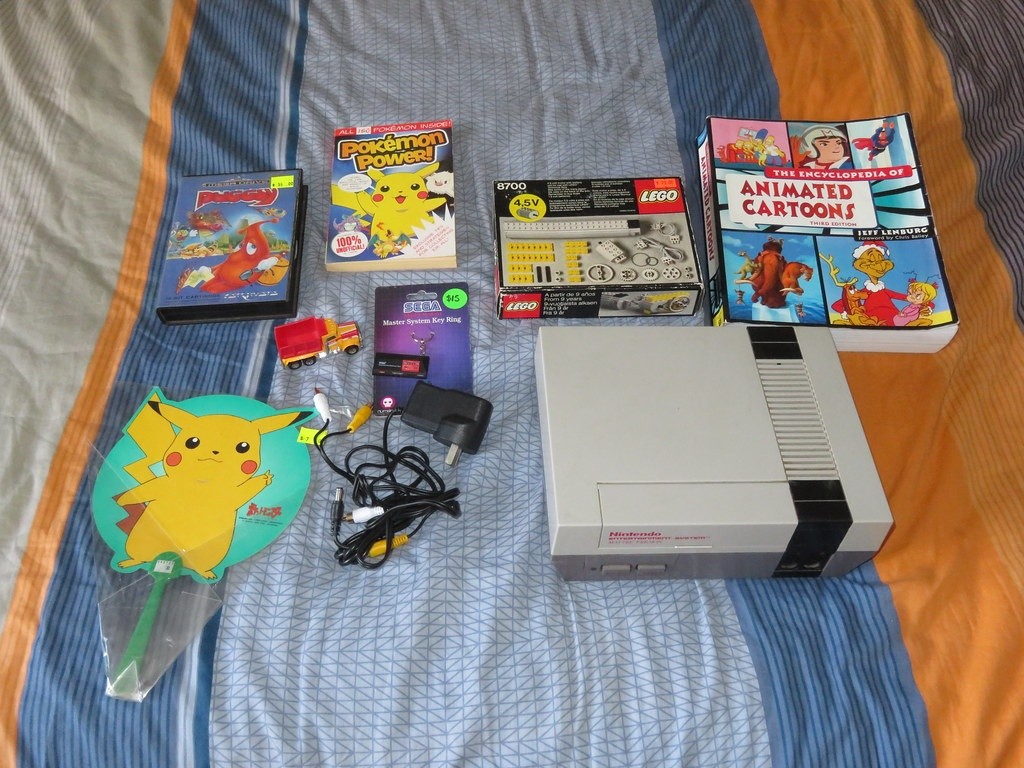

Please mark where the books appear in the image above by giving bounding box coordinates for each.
[693,111,960,353]
[325,120,458,271]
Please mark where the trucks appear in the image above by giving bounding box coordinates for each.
[274,314,363,369]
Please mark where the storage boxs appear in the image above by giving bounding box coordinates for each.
[490,176,704,320]
